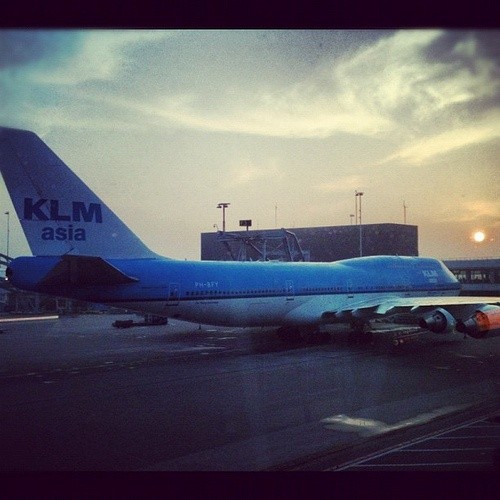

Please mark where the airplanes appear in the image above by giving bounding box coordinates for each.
[0,127,500,349]
